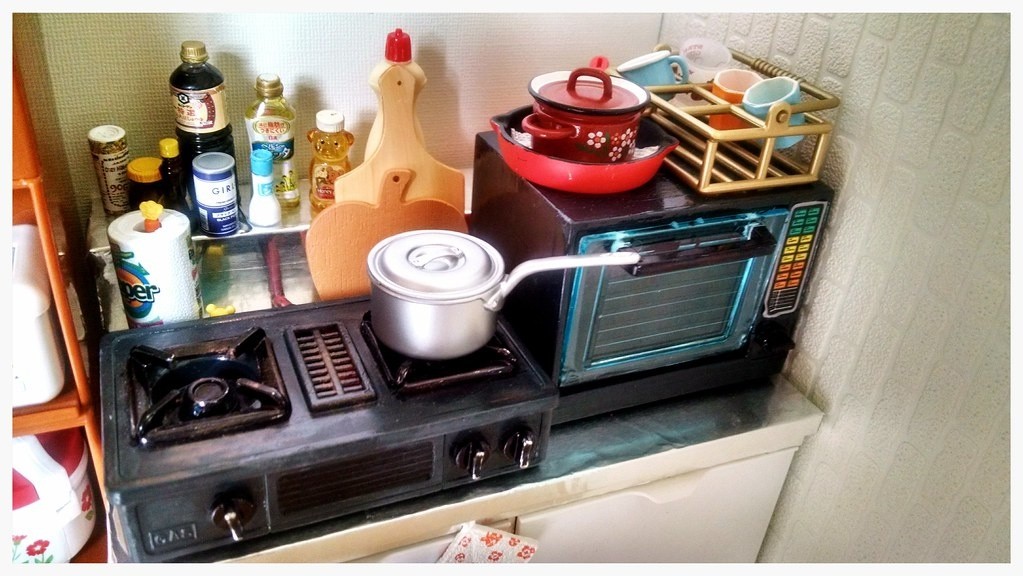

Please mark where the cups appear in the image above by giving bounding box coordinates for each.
[616,50,690,102]
[677,36,733,84]
[709,68,764,130]
[742,76,806,151]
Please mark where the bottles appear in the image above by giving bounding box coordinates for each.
[87,124,197,237]
[244,73,300,227]
[169,40,241,237]
[361,28,428,158]
[306,109,355,211]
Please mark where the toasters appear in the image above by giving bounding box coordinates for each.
[11,223,65,411]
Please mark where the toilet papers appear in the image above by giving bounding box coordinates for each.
[108,205,204,330]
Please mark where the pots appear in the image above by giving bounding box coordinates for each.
[490,104,679,194]
[522,56,651,163]
[365,229,641,359]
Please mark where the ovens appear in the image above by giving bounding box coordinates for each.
[470,129,835,428]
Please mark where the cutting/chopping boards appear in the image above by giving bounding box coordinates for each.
[333,64,465,221]
[305,169,468,301]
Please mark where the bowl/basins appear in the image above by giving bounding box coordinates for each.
[12,428,97,562]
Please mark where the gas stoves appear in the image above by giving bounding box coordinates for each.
[94,295,560,563]
